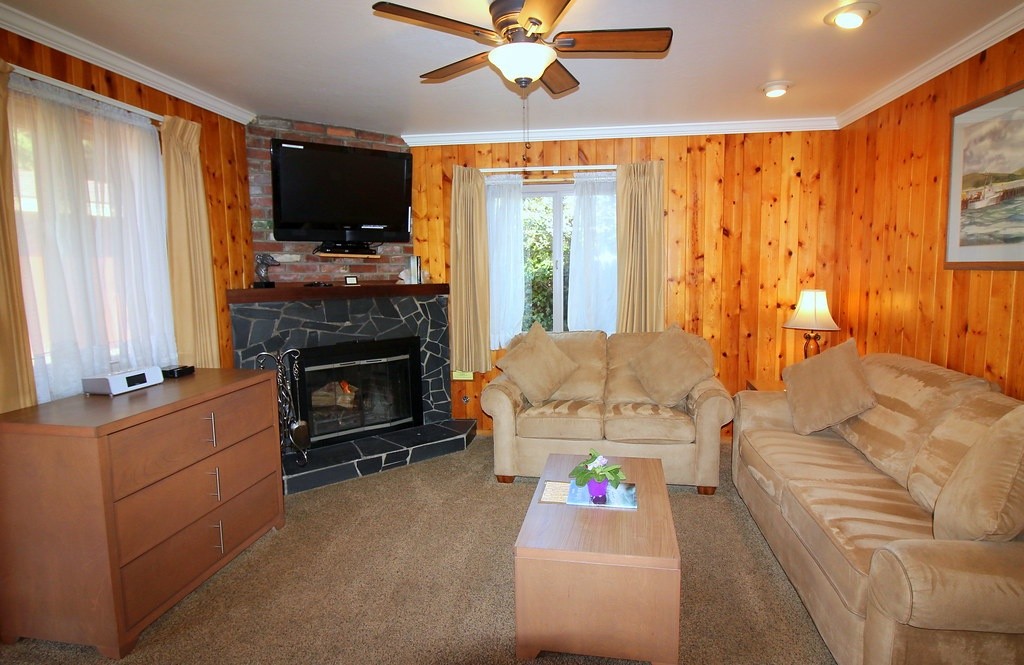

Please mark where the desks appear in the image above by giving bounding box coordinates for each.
[0,366,286,660]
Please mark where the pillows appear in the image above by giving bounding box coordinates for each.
[495,320,580,408]
[782,337,879,435]
[932,404,1024,543]
[629,323,715,408]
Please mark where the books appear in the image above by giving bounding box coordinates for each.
[410,256,421,284]
[567,480,637,509]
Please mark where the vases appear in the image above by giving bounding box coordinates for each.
[587,478,608,495]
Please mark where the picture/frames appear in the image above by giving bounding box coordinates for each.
[943,79,1024,272]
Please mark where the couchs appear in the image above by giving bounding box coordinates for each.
[480,329,736,495]
[731,353,1024,665]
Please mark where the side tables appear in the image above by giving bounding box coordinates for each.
[746,379,785,391]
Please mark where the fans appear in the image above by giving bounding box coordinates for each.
[372,0,673,95]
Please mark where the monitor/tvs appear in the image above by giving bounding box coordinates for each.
[270,138,412,254]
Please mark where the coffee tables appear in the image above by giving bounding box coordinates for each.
[514,453,682,665]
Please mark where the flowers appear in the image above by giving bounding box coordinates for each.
[568,448,627,489]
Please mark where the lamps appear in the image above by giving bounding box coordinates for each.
[823,2,882,30]
[488,32,558,162]
[781,289,841,359]
[759,80,793,98]
